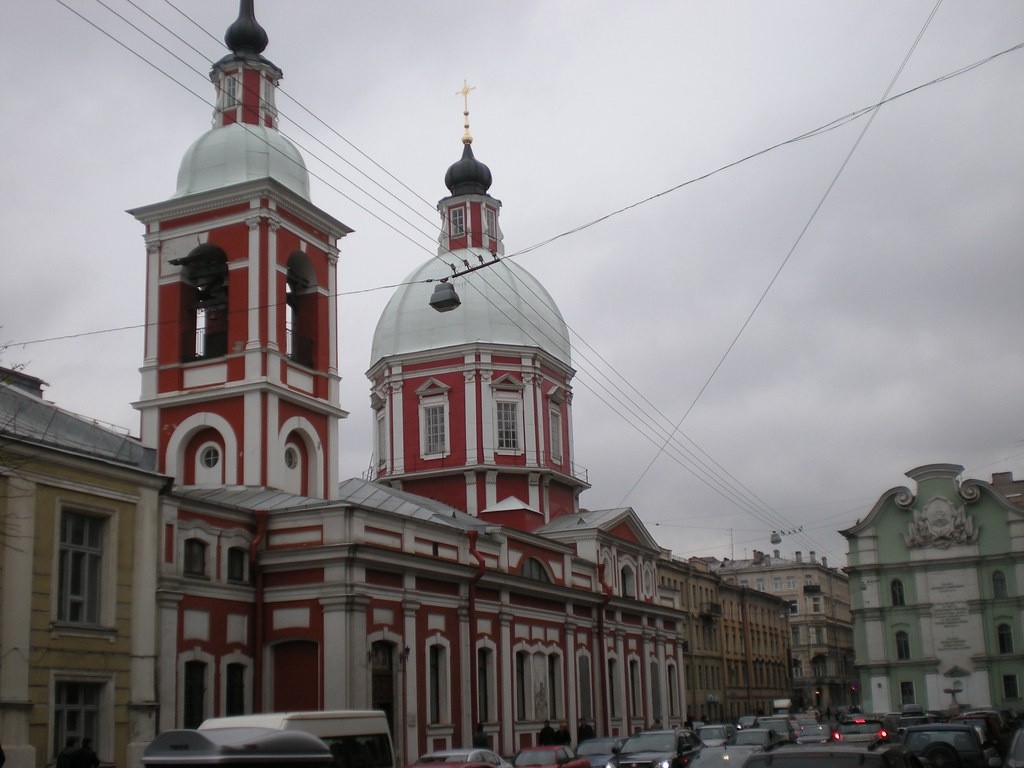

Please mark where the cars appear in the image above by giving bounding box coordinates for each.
[408,710,1024,767]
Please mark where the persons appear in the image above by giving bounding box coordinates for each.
[577,717,595,743]
[56,736,75,768]
[539,720,555,746]
[826,703,861,722]
[473,722,488,749]
[74,736,100,768]
[555,722,571,746]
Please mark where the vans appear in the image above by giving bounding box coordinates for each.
[198,710,396,768]
